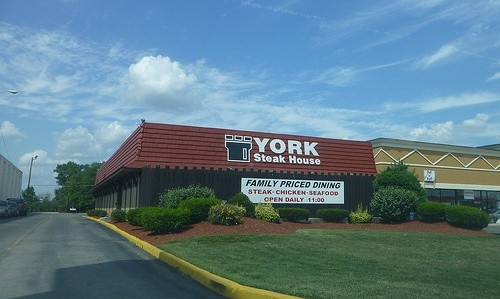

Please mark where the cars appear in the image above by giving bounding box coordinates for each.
[486,210,500,223]
[0,197,28,218]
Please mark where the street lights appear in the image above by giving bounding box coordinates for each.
[28,155,39,188]
